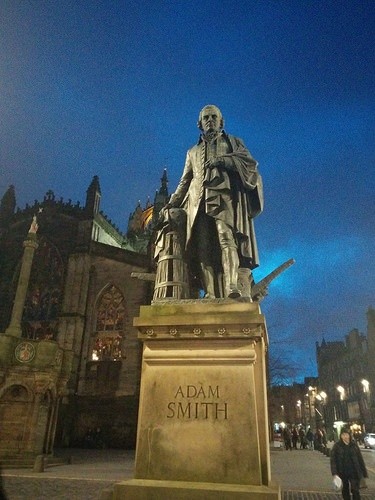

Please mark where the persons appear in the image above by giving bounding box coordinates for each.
[159,103,264,297]
[330,427,369,499]
[272,422,328,454]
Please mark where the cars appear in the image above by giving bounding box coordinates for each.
[364,433,375,449]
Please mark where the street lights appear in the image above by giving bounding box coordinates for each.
[279,380,370,431]
